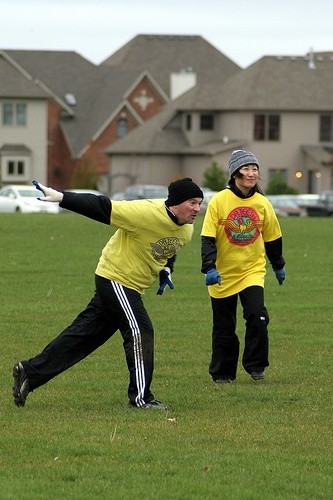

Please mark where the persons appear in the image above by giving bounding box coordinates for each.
[200,149,286,383]
[12,177,204,409]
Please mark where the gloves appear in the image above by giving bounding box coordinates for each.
[275,268,286,285]
[32,180,64,202]
[205,269,222,287]
[156,267,174,296]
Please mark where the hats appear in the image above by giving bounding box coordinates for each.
[228,149,259,175]
[166,177,203,207]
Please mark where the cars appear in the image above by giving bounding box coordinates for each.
[297,191,333,216]
[263,194,309,217]
[0,184,59,215]
[111,184,168,202]
[199,190,218,214]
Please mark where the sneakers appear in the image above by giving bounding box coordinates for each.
[128,400,167,410]
[215,378,232,383]
[251,371,265,381]
[12,362,30,408]
